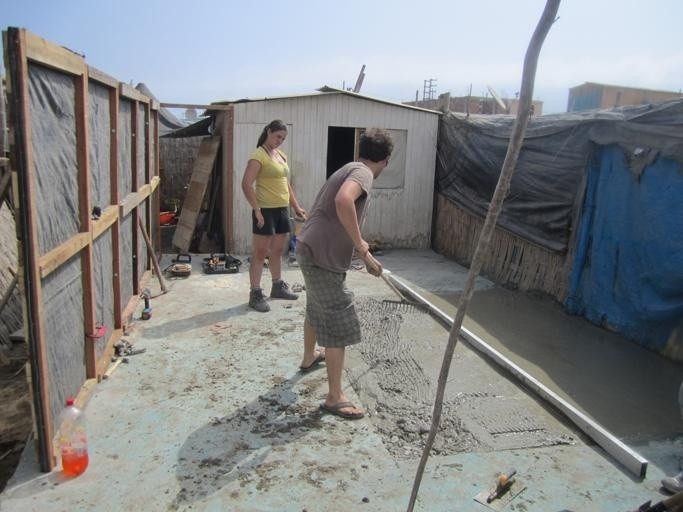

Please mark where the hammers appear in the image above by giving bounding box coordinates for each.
[103,357,128,378]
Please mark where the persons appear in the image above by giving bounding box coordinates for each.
[242,119,305,312]
[295,128,394,420]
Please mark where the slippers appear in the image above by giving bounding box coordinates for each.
[319,400,363,419]
[299,348,325,371]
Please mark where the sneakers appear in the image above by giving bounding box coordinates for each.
[249,288,270,311]
[270,280,297,299]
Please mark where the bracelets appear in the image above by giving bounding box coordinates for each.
[253,208,261,211]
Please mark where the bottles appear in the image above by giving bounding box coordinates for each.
[57,396,88,478]
